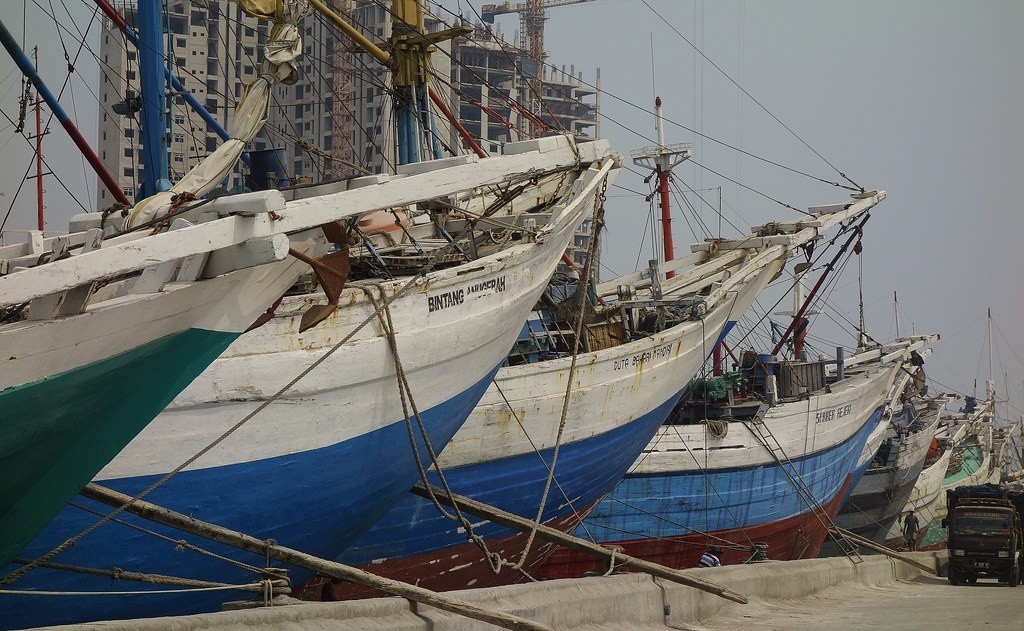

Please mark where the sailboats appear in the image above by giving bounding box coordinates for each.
[0,0,626,631]
[0,0,625,570]
[545,95,944,582]
[313,0,887,600]
[814,290,1024,559]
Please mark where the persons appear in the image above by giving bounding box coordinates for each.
[699,549,721,569]
[903,508,920,551]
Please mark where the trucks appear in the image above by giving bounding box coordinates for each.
[942,481,1024,587]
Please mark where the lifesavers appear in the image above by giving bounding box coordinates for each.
[926,437,937,459]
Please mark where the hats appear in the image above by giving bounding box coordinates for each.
[908,508,915,511]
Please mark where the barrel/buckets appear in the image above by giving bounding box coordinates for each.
[753,354,773,391]
